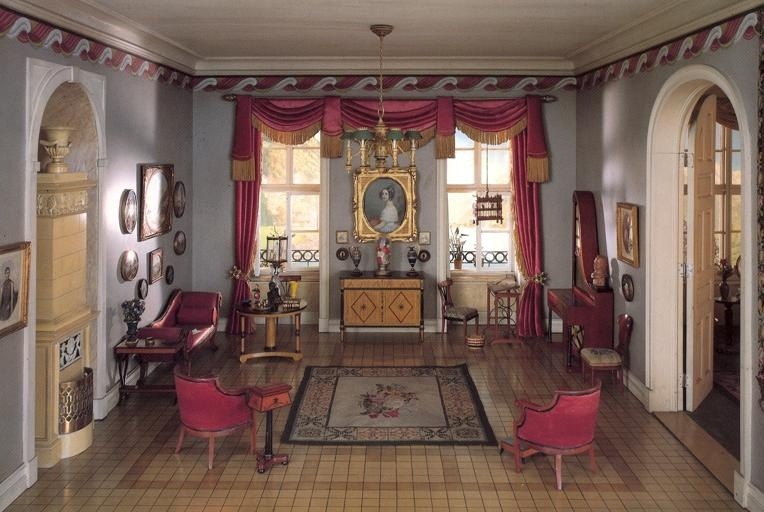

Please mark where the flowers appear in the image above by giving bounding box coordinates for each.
[121,297,146,321]
[716,258,737,280]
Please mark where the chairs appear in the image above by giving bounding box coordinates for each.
[579,313,634,393]
[438,278,480,344]
[497,385,603,489]
[171,369,255,471]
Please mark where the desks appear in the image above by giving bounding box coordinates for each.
[113,337,191,405]
[483,282,523,346]
[715,297,740,354]
[236,297,308,362]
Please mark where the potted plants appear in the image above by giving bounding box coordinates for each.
[447,227,469,269]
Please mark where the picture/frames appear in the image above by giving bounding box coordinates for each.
[0,241,31,337]
[352,170,416,243]
[119,162,187,298]
[615,202,640,269]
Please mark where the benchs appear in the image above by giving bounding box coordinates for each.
[134,289,221,367]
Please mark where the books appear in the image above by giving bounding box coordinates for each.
[282,296,301,308]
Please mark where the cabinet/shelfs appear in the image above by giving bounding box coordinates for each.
[547,190,615,373]
[338,271,425,343]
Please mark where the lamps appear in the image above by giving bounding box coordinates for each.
[342,22,419,173]
[266,235,288,299]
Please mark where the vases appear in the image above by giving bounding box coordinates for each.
[719,280,731,299]
[126,319,140,335]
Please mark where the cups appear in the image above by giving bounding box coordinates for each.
[289,281,298,299]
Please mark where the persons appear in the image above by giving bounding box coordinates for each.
[0,268,17,315]
[622,281,629,297]
[372,186,401,234]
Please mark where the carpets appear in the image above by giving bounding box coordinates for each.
[712,369,740,405]
[281,364,501,448]
